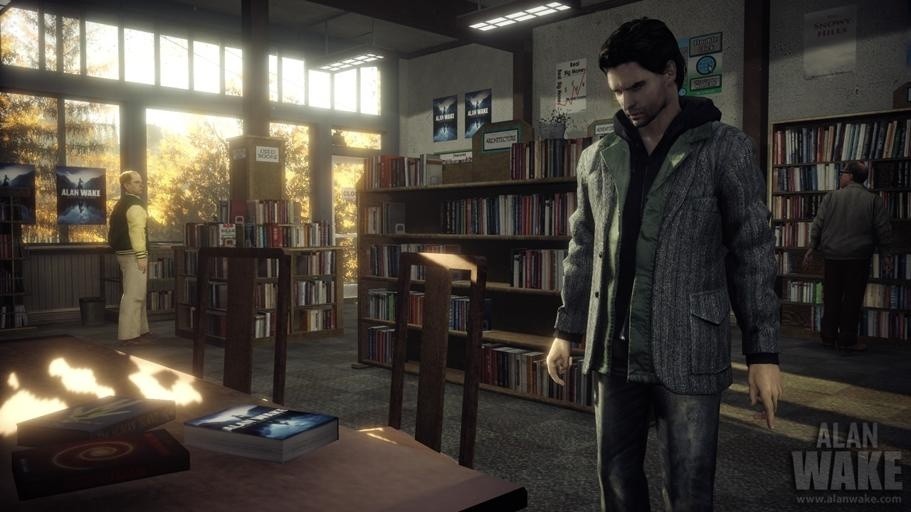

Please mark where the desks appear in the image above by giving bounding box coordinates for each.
[0,334,527,512]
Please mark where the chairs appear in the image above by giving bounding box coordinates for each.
[356,252,489,469]
[193,247,292,407]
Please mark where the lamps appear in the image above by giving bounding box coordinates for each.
[457,0,572,32]
[308,18,388,74]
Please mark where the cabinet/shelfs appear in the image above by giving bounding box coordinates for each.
[768,108,911,346]
[171,222,345,346]
[99,244,176,325]
[0,186,38,342]
[355,134,609,413]
